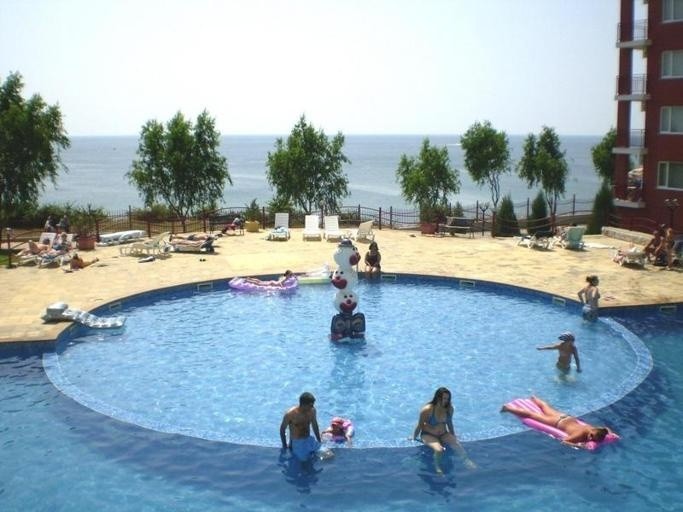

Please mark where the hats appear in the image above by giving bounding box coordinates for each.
[558,332,574,342]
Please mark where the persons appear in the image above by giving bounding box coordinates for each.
[614,222,675,268]
[364,242,381,280]
[500,395,619,449]
[536,331,582,374]
[164,215,259,245]
[244,270,293,287]
[321,416,352,447]
[279,392,320,462]
[14,214,99,270]
[578,275,601,321]
[413,387,474,473]
[559,222,577,243]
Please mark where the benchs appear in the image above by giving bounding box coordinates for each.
[434,216,475,237]
[206,213,245,234]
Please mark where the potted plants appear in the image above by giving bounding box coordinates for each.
[417,199,438,236]
[71,203,104,249]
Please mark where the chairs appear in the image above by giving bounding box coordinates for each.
[551,226,586,249]
[609,232,682,272]
[23,231,217,267]
[514,228,560,249]
[267,208,377,242]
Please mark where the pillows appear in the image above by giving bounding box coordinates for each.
[44,301,67,314]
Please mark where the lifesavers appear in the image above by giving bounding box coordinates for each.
[321,419,354,443]
